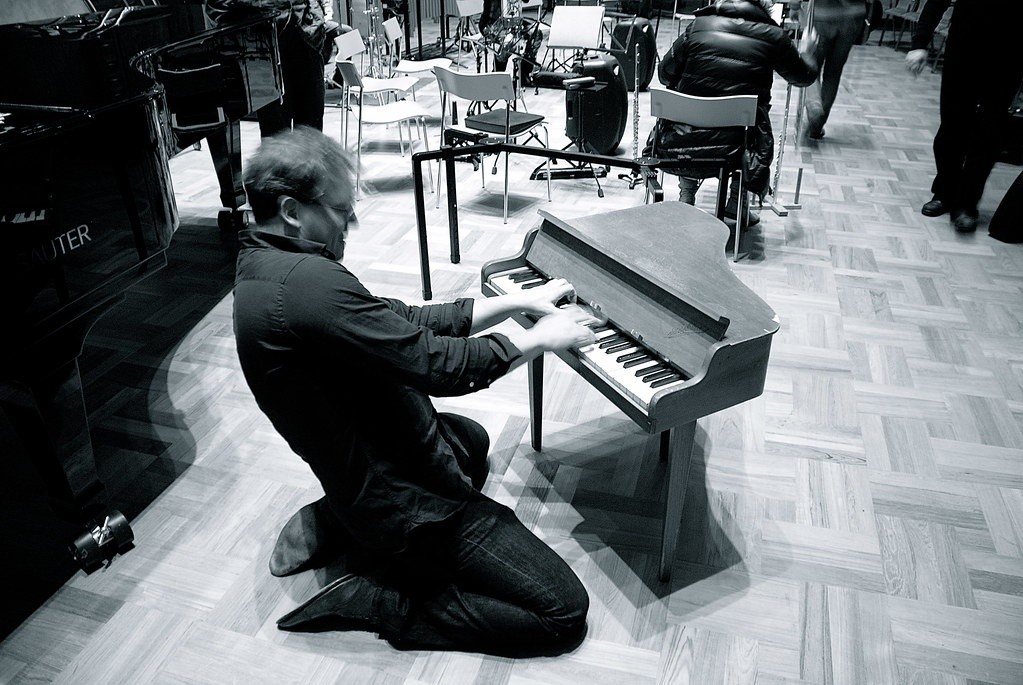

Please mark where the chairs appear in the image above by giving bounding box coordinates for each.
[334,0,553,224]
[644,87,759,263]
[879,0,956,74]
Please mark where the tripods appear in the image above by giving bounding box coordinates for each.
[619,44,645,189]
[528,83,608,196]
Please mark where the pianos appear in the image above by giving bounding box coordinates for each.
[0,0,288,576]
[480,199,782,584]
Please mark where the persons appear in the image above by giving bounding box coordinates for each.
[232,125,600,657]
[205,0,326,140]
[656,0,1023,232]
[320,0,353,85]
[479,0,543,86]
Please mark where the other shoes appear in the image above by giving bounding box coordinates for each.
[922,200,950,217]
[805,113,825,140]
[955,209,977,233]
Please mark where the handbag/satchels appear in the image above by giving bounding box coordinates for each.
[809,3,871,45]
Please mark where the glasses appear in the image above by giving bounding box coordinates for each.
[315,200,356,217]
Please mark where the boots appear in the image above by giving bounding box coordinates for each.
[268,494,366,577]
[724,180,761,223]
[677,176,699,206]
[275,570,408,629]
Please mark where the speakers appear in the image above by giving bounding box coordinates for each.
[564,53,632,154]
[612,17,658,91]
